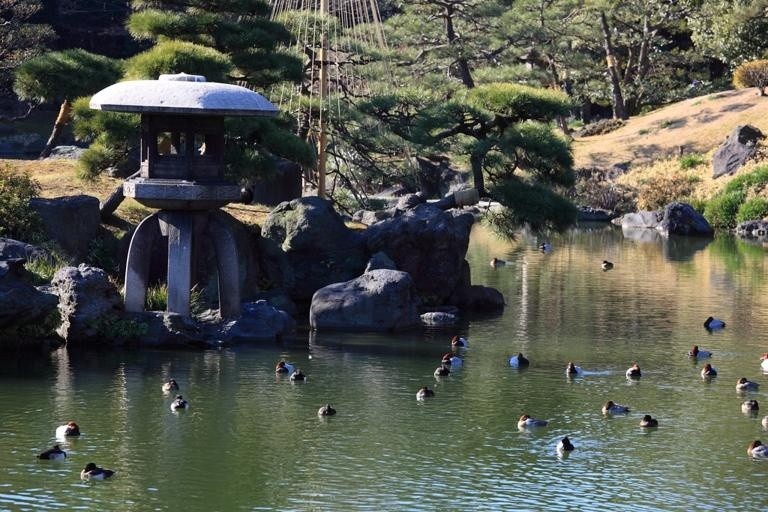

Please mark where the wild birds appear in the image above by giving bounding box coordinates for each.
[275,361,307,381]
[318,403,336,416]
[507,353,529,369]
[161,378,189,413]
[602,261,613,271]
[566,362,577,377]
[37,422,115,480]
[625,360,641,378]
[517,414,574,452]
[538,242,552,253]
[602,401,659,429]
[736,377,768,459]
[416,336,466,398]
[490,258,505,268]
[687,317,725,381]
[760,352,768,375]
